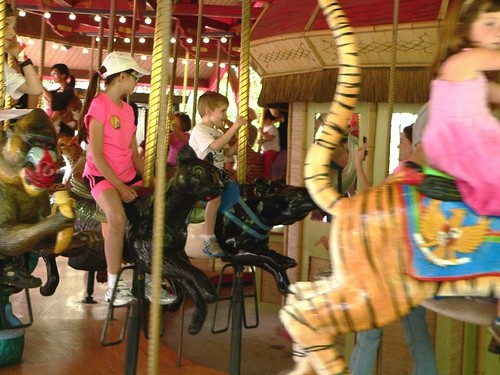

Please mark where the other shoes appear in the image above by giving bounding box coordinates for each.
[488,322,500,353]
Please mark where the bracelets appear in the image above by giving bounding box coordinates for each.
[20,59,32,67]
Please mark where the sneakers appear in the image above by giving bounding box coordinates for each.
[143,281,179,304]
[104,279,139,306]
[202,237,225,257]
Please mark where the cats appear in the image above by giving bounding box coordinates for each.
[39,146,225,333]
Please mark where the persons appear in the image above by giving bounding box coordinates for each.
[39,62,81,137]
[259,108,287,183]
[0,5,43,289]
[77,51,178,307]
[412,0,500,356]
[137,111,191,166]
[189,92,258,260]
[351,121,440,375]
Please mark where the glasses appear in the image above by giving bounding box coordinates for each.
[124,71,139,83]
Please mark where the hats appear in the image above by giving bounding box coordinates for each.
[100,51,151,79]
